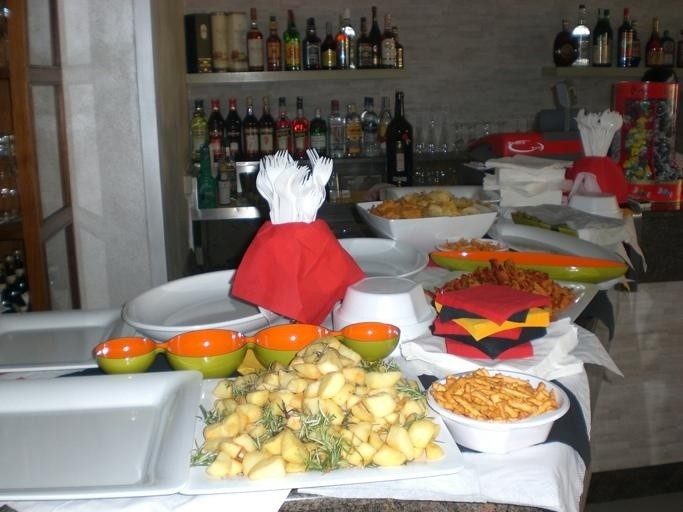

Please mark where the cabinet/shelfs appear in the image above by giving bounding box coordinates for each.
[0,0,82,313]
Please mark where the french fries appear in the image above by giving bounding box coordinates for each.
[429,366,560,424]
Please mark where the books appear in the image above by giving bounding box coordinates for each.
[510,204,629,247]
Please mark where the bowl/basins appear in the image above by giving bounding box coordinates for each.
[358,196,496,246]
[337,322,399,366]
[160,330,251,379]
[250,323,335,375]
[119,269,286,344]
[94,337,158,373]
[335,277,430,327]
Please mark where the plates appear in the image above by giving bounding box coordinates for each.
[490,219,626,264]
[328,306,436,341]
[0,309,135,373]
[426,371,570,450]
[159,374,466,482]
[328,238,431,279]
[429,267,598,327]
[0,374,180,497]
[434,248,633,283]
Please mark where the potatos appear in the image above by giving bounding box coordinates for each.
[204,336,443,481]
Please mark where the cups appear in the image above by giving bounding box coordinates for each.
[409,116,507,190]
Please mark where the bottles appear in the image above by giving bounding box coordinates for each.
[181,88,408,197]
[0,134,26,312]
[546,5,682,66]
[247,7,405,71]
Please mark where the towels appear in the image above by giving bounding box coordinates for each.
[429,285,551,362]
